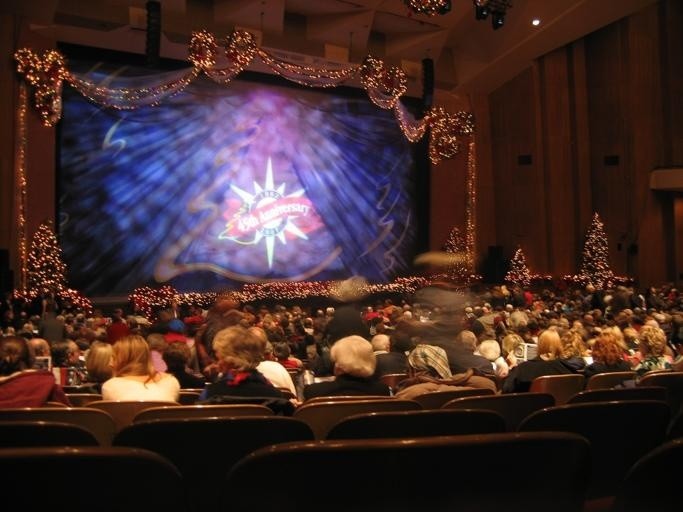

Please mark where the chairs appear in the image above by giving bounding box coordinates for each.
[0,373,682,512]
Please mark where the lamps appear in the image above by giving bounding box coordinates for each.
[439,0,452,15]
[475,4,490,21]
[492,8,505,31]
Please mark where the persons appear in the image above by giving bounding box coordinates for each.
[217,302,262,328]
[395,344,465,393]
[289,302,332,378]
[533,288,569,339]
[248,327,296,397]
[500,334,526,367]
[371,335,406,376]
[624,327,641,353]
[77,342,113,394]
[334,300,438,341]
[568,285,602,341]
[602,284,683,331]
[438,293,533,335]
[302,334,391,397]
[481,340,509,376]
[0,310,90,407]
[162,341,205,389]
[633,328,675,384]
[262,303,289,360]
[582,340,630,390]
[103,336,181,404]
[446,331,492,380]
[148,301,210,341]
[90,307,147,344]
[196,334,285,413]
[498,330,576,394]
